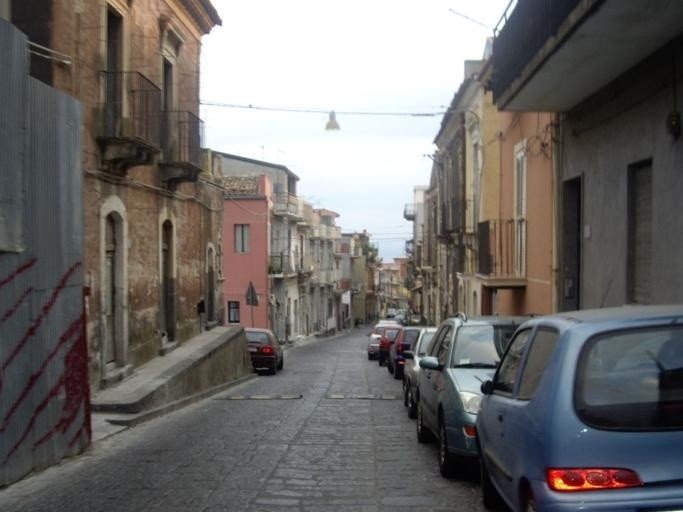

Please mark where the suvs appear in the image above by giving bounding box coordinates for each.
[414,308,541,480]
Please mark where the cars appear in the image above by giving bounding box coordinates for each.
[474,301,683,512]
[366,307,438,407]
[241,325,284,376]
